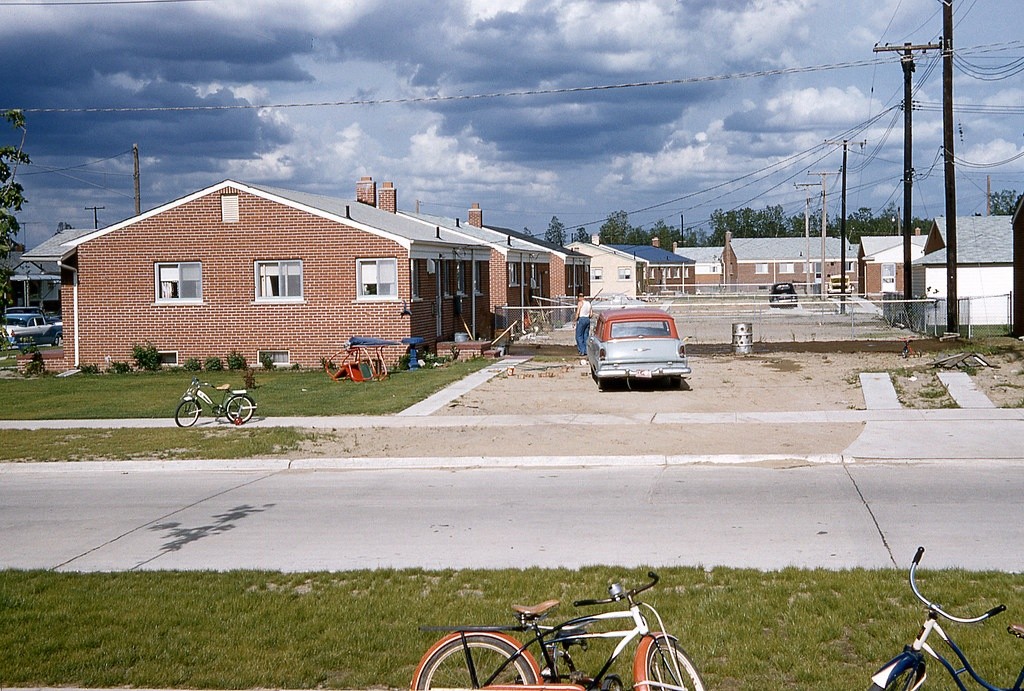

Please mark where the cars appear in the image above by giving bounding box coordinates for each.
[588,309,691,391]
[0,313,63,348]
[768,283,799,308]
[4,307,63,322]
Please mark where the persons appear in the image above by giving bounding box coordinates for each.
[574,292,594,356]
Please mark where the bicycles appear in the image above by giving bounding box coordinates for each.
[867,546,1024,691]
[175,377,258,428]
[409,571,706,691]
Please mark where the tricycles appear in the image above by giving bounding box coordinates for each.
[897,336,923,359]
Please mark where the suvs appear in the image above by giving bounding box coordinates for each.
[827,274,852,299]
